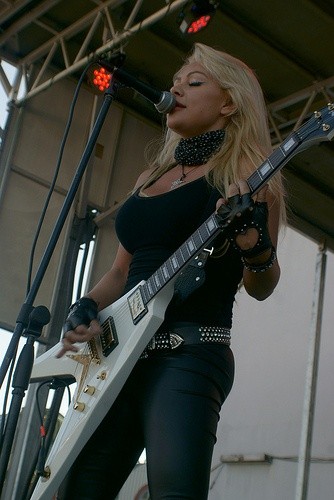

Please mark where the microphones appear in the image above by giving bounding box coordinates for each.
[98,59,176,114]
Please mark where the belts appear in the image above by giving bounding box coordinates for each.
[139,326,231,359]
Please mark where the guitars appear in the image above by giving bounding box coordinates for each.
[27,101,334,500]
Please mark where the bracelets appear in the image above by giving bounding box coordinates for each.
[241,246,277,274]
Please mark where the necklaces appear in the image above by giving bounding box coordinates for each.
[171,164,199,188]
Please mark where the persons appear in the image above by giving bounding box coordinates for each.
[55,43,289,500]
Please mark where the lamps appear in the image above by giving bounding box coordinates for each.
[79,63,112,95]
[176,0,216,35]
